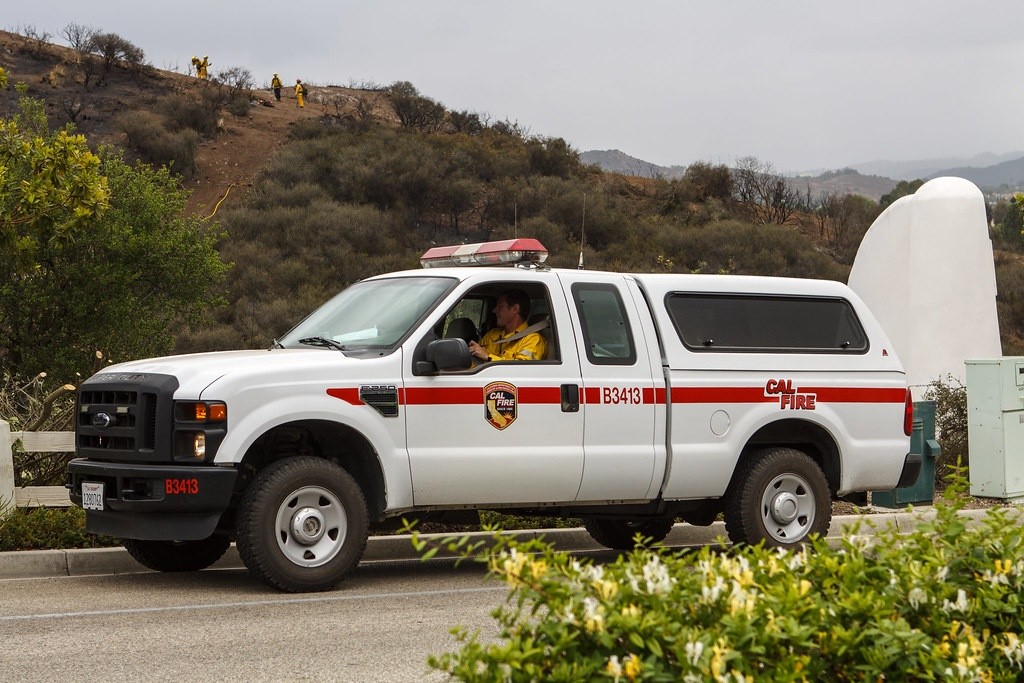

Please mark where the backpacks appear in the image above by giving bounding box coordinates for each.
[302,89,307,95]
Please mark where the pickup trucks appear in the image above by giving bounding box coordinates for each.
[65,237,913,593]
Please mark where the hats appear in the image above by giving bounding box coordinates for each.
[297,79,301,82]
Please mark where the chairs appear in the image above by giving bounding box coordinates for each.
[527,312,558,361]
[444,317,481,347]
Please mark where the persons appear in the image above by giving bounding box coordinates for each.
[295,79,304,108]
[271,74,282,102]
[192,55,212,79]
[468,289,548,370]
